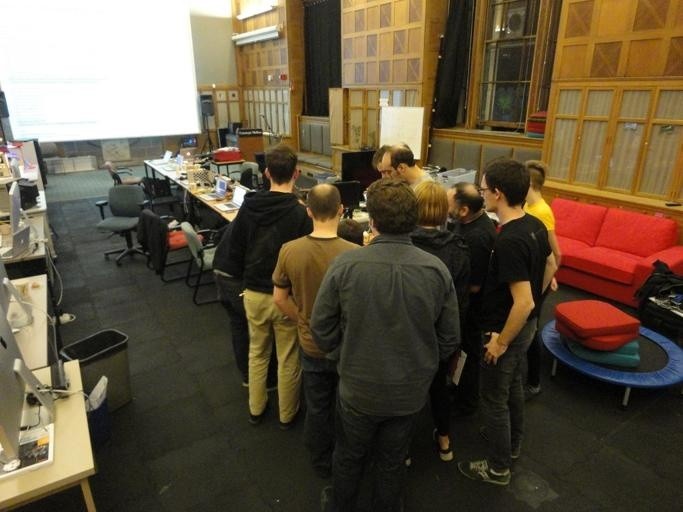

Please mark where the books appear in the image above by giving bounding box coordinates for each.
[0,423,54,476]
[435,167,477,191]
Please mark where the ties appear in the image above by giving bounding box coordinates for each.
[10,181,31,233]
[0,261,34,328]
[0,307,56,457]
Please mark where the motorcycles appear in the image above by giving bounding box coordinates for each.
[199,116,215,154]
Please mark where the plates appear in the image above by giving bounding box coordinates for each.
[586,194,641,215]
[568,83,657,196]
[634,82,682,204]
[179,84,421,178]
[538,186,588,209]
[539,83,588,186]
[639,204,682,246]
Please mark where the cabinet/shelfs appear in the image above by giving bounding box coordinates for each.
[179,84,421,178]
[538,186,588,209]
[539,83,588,186]
[639,204,682,246]
[568,83,657,196]
[634,82,682,204]
[586,194,641,215]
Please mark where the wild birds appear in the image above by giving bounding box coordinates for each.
[152,150,171,164]
[199,179,228,201]
[162,155,184,171]
[1,226,32,260]
[214,184,251,211]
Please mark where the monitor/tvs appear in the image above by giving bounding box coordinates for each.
[0,423,54,476]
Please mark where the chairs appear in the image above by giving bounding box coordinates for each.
[434,435,453,461]
[293,173,377,245]
[522,384,541,401]
[319,485,332,510]
[248,397,270,424]
[91,143,265,305]
[512,437,524,458]
[458,458,511,486]
[278,405,302,431]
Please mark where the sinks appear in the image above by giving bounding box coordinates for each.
[541,196,682,310]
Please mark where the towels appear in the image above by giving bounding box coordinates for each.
[58,329,133,414]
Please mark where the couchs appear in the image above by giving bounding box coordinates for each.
[541,196,682,310]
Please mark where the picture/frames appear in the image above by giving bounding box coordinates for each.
[0,139,103,511]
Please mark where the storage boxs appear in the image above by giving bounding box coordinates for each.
[435,167,477,191]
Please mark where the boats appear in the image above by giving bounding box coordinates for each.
[200,95,215,116]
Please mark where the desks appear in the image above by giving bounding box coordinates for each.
[0,139,103,511]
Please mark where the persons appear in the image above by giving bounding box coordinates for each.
[211,145,313,431]
[337,219,365,247]
[273,184,363,479]
[374,142,433,197]
[458,154,558,485]
[476,155,560,401]
[411,180,496,460]
[311,178,461,511]
